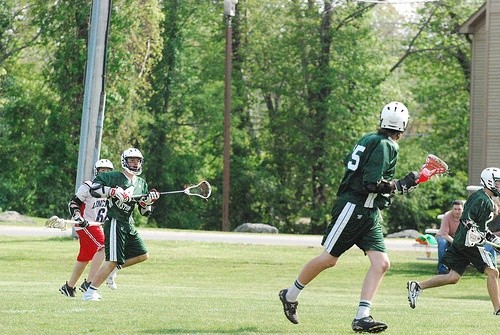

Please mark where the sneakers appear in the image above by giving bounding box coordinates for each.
[83,293,99,301]
[279,289,298,324]
[106,276,117,289]
[407,280,421,308]
[79,279,92,292]
[59,281,76,297]
[352,316,388,333]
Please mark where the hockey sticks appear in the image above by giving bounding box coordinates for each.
[465,227,500,248]
[130,181,212,199]
[45,216,105,231]
[415,153,449,183]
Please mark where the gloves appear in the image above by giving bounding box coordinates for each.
[393,170,420,195]
[489,234,500,253]
[114,187,131,205]
[142,188,160,205]
[75,214,89,227]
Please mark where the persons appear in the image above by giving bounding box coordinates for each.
[59,158,114,298]
[279,101,422,332]
[83,146,160,301]
[435,199,463,274]
[483,196,500,264]
[407,167,500,315]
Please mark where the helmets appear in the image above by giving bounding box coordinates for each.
[379,101,410,131]
[121,148,143,175]
[480,167,500,196]
[93,158,114,176]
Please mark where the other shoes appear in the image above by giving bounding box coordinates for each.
[439,269,448,274]
